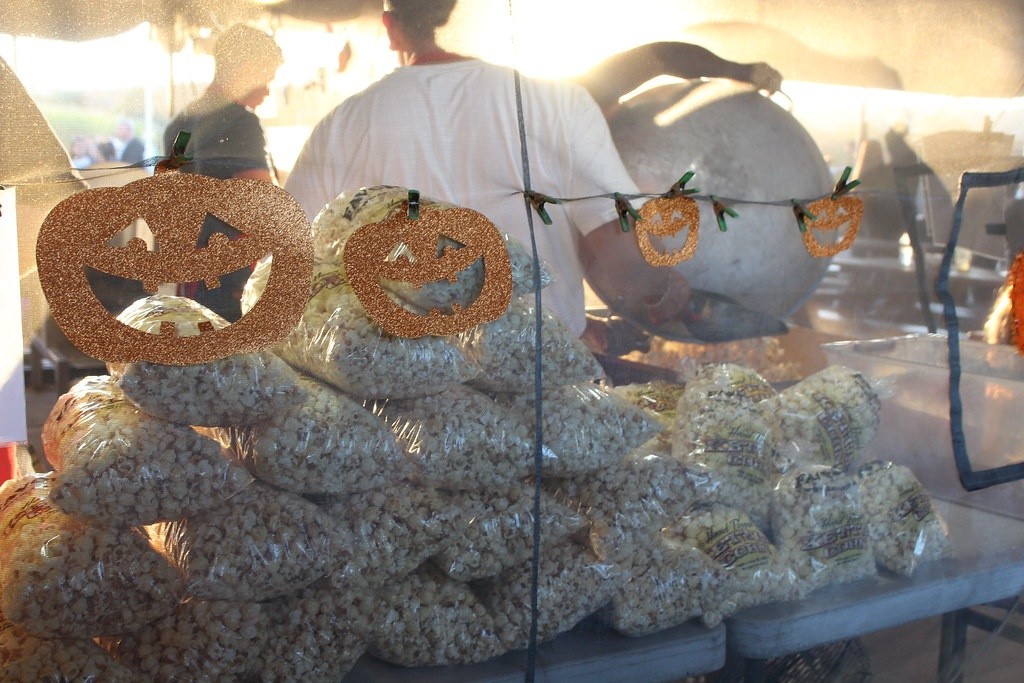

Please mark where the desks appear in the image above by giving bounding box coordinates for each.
[357,307,1024,683]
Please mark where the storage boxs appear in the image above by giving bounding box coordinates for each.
[817,331,1023,521]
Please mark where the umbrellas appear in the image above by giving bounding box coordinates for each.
[0,0,387,114]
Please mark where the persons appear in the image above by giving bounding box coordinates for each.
[281,0,682,366]
[158,25,284,324]
[560,41,786,356]
[832,129,919,323]
[64,118,145,170]
